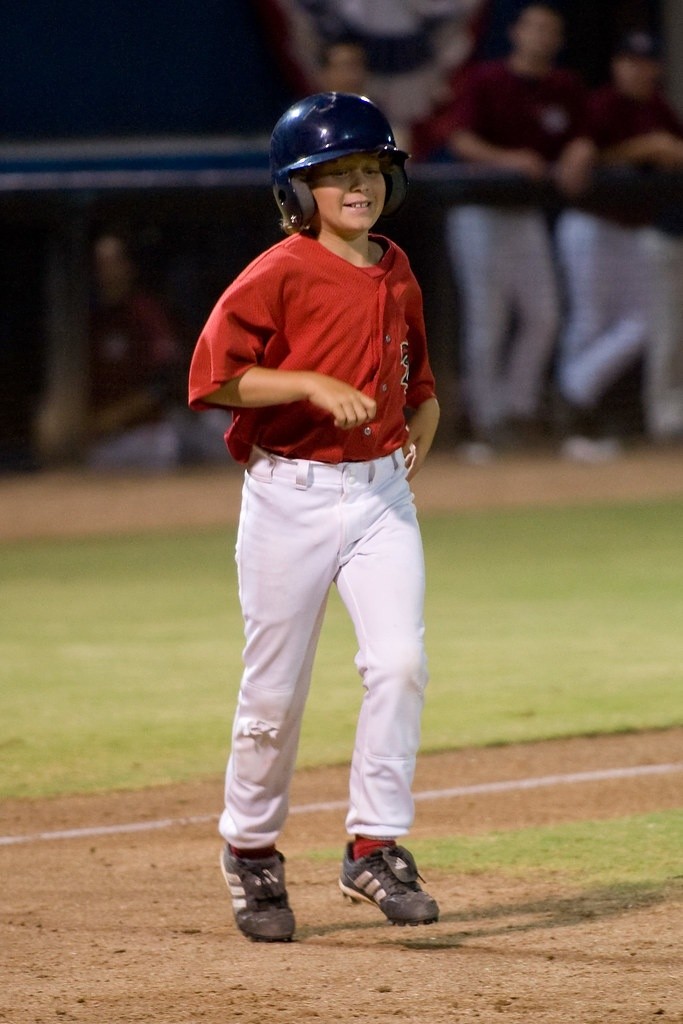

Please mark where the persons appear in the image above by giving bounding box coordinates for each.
[35,233,189,471]
[558,28,683,443]
[309,32,364,102]
[188,93,443,942]
[433,0,595,461]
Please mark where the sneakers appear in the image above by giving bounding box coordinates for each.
[337,839,441,927]
[218,842,297,942]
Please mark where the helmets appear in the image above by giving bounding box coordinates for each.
[268,91,413,233]
